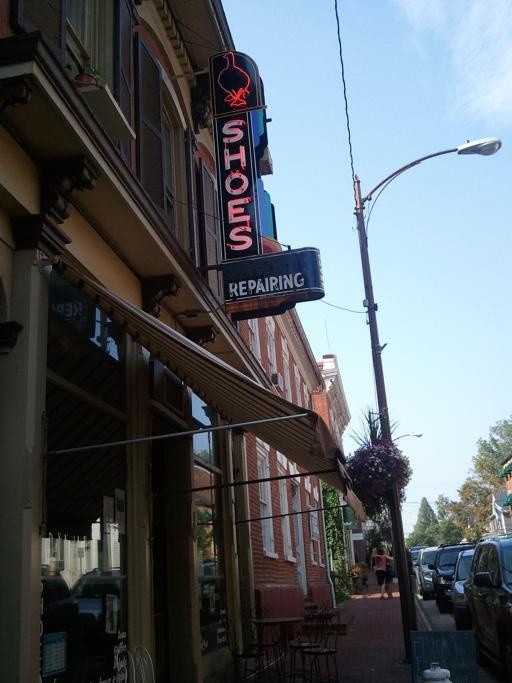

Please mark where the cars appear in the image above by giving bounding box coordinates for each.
[42,559,224,682]
[404,535,510,673]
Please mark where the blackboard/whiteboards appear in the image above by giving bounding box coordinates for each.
[410,630,479,683]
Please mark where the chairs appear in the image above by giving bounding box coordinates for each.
[226,623,264,683]
[304,613,341,683]
[289,606,328,683]
[248,605,279,683]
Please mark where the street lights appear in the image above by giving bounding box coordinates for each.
[392,430,423,444]
[350,133,500,672]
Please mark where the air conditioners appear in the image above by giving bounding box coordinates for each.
[270,373,283,391]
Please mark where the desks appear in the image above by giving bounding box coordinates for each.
[253,617,304,682]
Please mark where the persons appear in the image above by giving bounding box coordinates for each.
[368,541,413,600]
